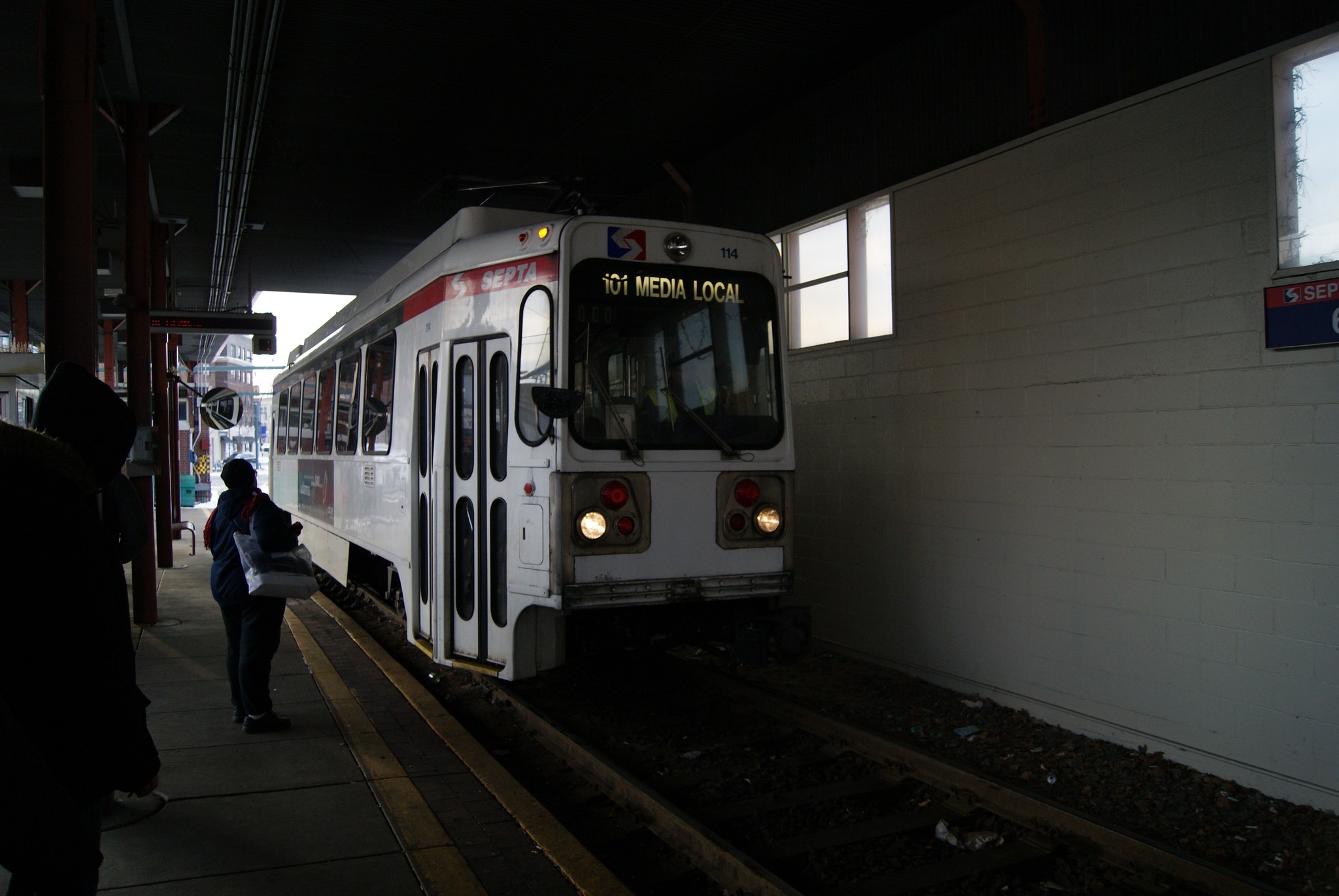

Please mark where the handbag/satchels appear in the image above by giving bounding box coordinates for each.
[233,510,320,600]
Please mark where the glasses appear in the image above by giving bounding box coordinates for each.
[255,470,257,479]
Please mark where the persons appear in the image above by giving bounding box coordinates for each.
[642,350,722,427]
[0,414,124,895]
[20,364,170,829]
[203,457,304,739]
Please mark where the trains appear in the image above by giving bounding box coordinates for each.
[267,206,795,682]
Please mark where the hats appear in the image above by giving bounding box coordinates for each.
[220,459,255,489]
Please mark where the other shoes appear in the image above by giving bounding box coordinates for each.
[230,709,291,733]
